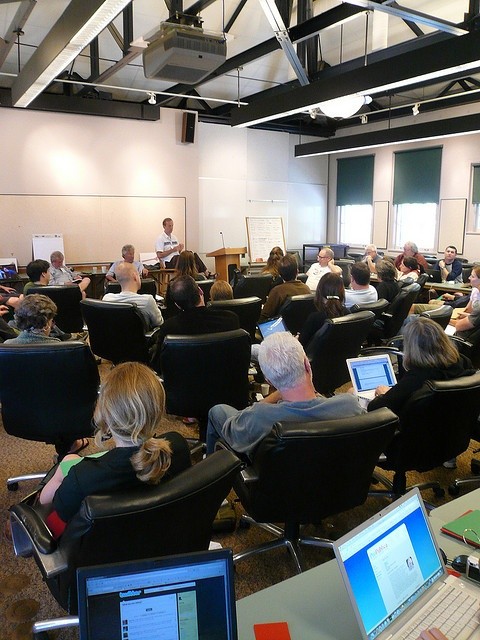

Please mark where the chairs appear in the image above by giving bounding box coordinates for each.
[10,450,243,638]
[380,282,421,343]
[236,274,274,299]
[24,285,84,330]
[273,293,317,328]
[333,261,350,286]
[2,339,100,498]
[206,296,260,336]
[355,299,390,325]
[450,455,480,496]
[392,276,413,301]
[450,293,472,312]
[386,326,474,356]
[158,331,251,456]
[106,278,156,305]
[417,273,429,292]
[195,279,215,299]
[357,351,480,510]
[228,407,399,571]
[424,301,454,331]
[302,309,373,394]
[79,297,157,364]
[278,273,307,287]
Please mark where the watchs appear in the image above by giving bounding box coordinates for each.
[440,266,447,270]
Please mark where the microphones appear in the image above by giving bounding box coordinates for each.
[220,231,223,234]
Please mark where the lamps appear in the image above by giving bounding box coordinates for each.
[320,91,373,121]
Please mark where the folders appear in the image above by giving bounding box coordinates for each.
[440,510,480,548]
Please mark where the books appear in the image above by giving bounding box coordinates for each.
[440,509,480,550]
[367,315,476,416]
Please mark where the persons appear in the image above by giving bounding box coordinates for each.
[0,284,25,313]
[449,264,480,327]
[415,627,448,639]
[38,361,192,525]
[305,247,344,295]
[253,254,313,334]
[342,260,379,311]
[105,245,149,283]
[22,259,51,296]
[361,244,384,274]
[47,251,91,331]
[209,279,234,301]
[392,241,428,276]
[1,292,88,345]
[299,271,351,346]
[155,217,221,279]
[412,292,472,316]
[148,273,238,376]
[205,333,370,464]
[166,250,207,298]
[259,246,284,283]
[395,256,427,288]
[432,245,464,284]
[0,304,19,344]
[375,260,403,302]
[455,305,480,332]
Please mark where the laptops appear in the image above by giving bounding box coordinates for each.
[334,489,480,636]
[76,545,237,640]
[0,265,24,283]
[346,356,398,399]
[258,318,288,339]
[427,270,442,282]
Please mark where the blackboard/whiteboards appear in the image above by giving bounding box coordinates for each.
[0,194,187,269]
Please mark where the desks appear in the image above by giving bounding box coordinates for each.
[369,275,471,294]
[234,530,480,638]
[422,483,480,577]
